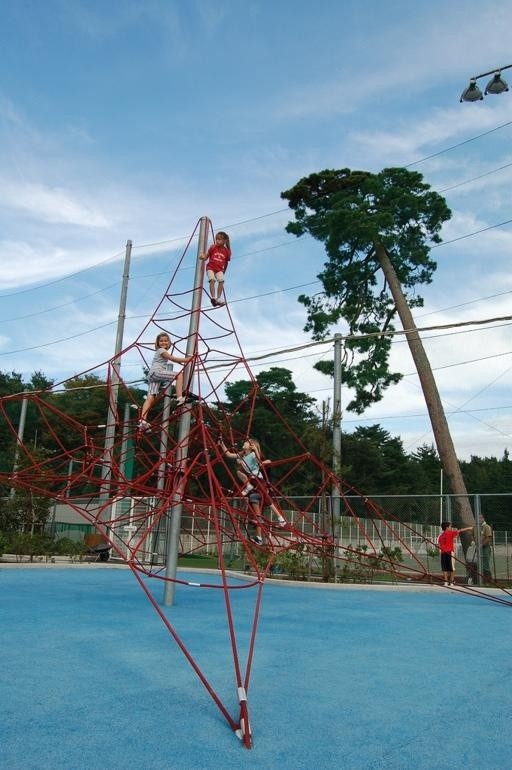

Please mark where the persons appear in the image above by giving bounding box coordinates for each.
[139,331,196,434]
[199,233,230,305]
[472,514,494,587]
[215,437,286,545]
[438,521,475,586]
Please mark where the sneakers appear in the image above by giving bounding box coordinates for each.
[249,535,264,546]
[138,419,152,432]
[240,483,254,496]
[274,520,288,528]
[444,580,455,587]
[174,396,190,407]
[210,296,226,307]
